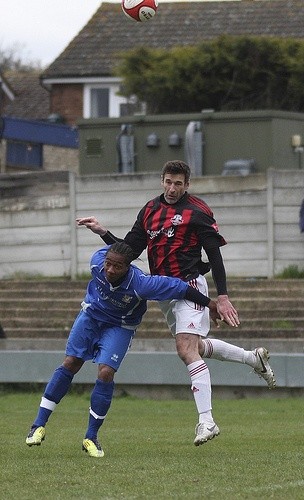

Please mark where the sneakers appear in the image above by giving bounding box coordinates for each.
[248,346,276,390]
[82,436,105,458]
[193,417,220,446]
[26,424,46,447]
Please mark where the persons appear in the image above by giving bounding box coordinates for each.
[25,242,231,458]
[74,159,276,446]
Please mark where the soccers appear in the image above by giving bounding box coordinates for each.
[121,0,159,23]
